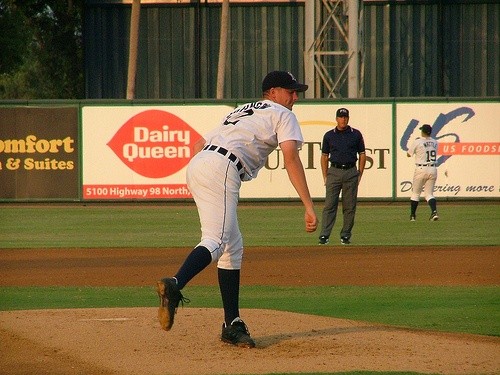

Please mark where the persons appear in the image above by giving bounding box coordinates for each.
[318,108,366,245]
[407,124,439,221]
[154,71,319,349]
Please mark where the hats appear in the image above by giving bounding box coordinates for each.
[262,71,308,93]
[336,108,349,117]
[419,125,432,134]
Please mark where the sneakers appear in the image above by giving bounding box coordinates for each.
[429,210,438,221]
[409,215,416,222]
[319,235,329,245]
[221,317,255,349]
[340,236,350,244]
[154,277,190,331]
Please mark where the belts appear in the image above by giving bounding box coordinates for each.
[419,164,434,166]
[331,163,355,170]
[204,145,245,180]
